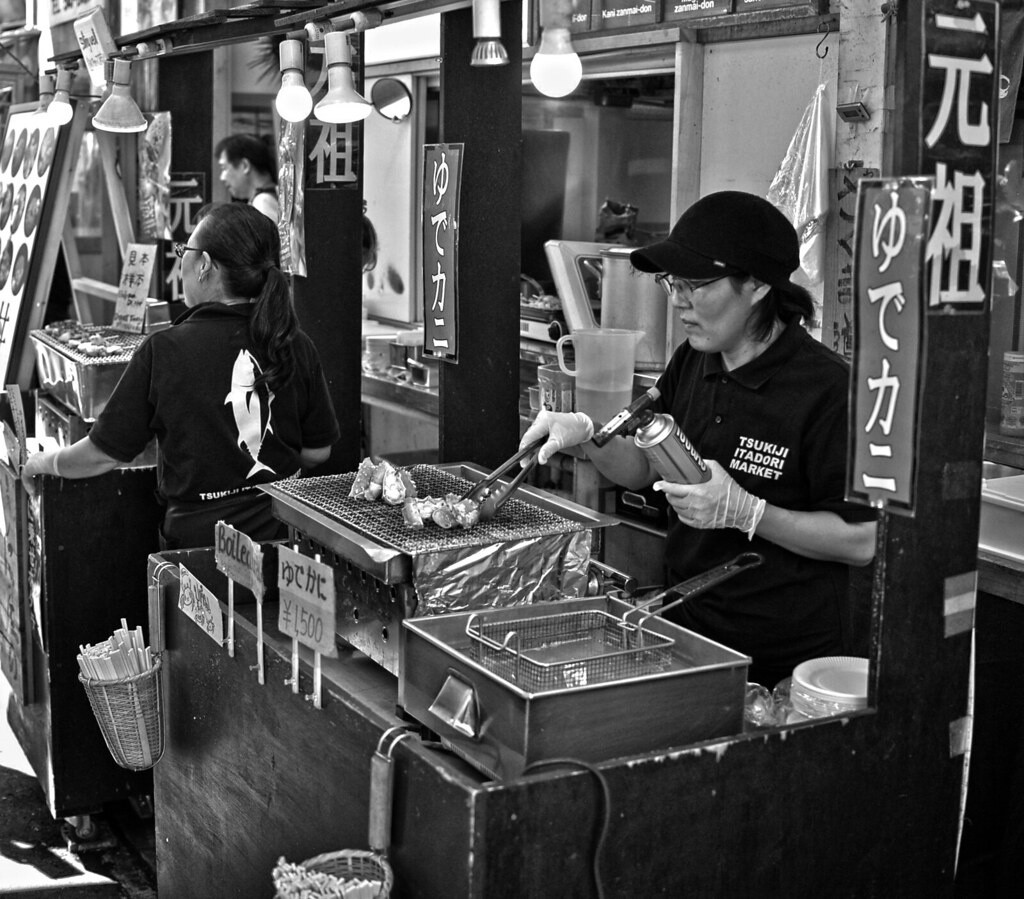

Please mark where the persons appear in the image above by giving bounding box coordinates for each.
[518,192,877,686]
[22,133,378,552]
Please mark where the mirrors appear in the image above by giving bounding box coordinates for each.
[371,79,414,122]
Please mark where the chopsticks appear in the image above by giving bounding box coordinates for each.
[271,856,382,899]
[77,618,152,680]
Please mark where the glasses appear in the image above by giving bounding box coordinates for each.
[175,241,219,271]
[654,272,731,300]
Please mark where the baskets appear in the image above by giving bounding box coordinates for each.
[275,849,411,899]
[78,651,176,771]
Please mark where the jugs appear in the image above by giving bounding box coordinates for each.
[556,327,646,428]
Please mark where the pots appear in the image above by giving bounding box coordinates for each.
[598,247,666,371]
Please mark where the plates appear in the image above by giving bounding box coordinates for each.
[786,656,870,725]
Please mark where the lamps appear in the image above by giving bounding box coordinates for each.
[313,8,394,125]
[91,38,173,133]
[273,20,334,122]
[27,58,85,131]
[530,0,587,98]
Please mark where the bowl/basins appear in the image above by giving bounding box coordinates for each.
[388,340,428,367]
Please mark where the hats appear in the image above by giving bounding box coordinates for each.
[630,191,800,286]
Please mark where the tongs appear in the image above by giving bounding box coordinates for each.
[459,433,549,523]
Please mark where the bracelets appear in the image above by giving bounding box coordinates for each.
[53,448,67,477]
